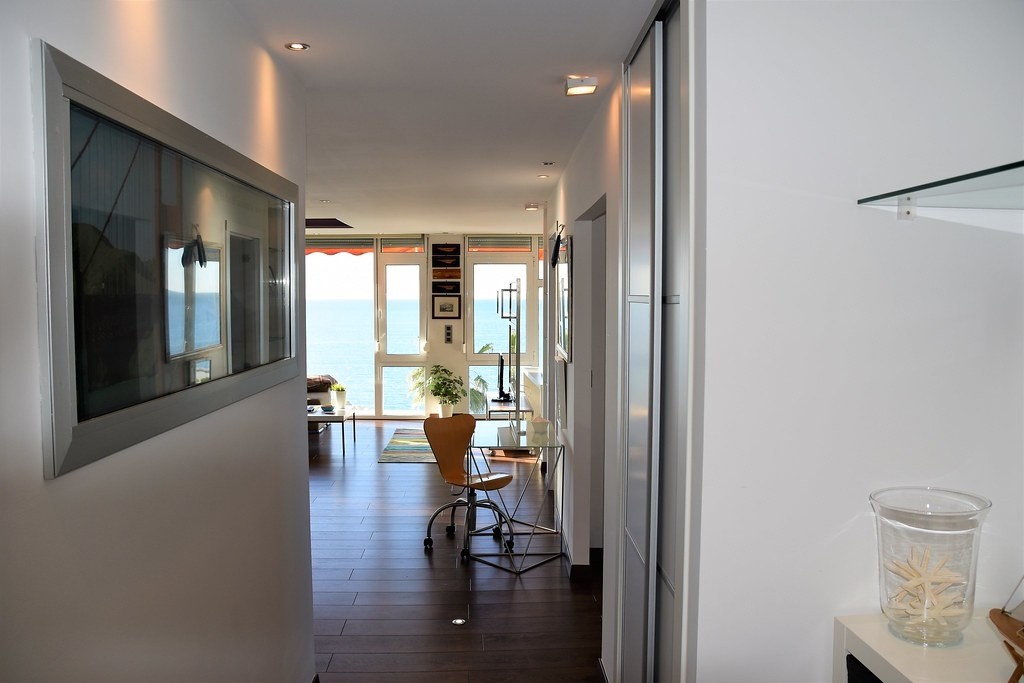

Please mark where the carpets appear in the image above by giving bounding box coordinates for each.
[378,428,437,464]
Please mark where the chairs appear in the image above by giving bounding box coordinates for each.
[423,416,515,551]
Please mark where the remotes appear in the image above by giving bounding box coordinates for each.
[307,407,314,412]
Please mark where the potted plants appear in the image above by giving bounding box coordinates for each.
[331,383,347,408]
[428,363,468,419]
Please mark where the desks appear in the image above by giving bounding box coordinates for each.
[486,392,535,421]
[466,421,566,577]
[306,405,357,459]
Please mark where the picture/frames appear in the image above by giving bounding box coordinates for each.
[432,294,461,319]
[555,234,571,362]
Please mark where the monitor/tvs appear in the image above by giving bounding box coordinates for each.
[491,353,512,402]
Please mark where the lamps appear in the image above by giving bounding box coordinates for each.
[565,77,600,96]
[525,205,539,211]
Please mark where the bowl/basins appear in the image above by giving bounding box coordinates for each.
[321,406,335,411]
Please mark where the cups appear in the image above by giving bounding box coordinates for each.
[870,486,993,648]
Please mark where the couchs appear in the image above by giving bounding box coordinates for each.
[306,374,338,434]
